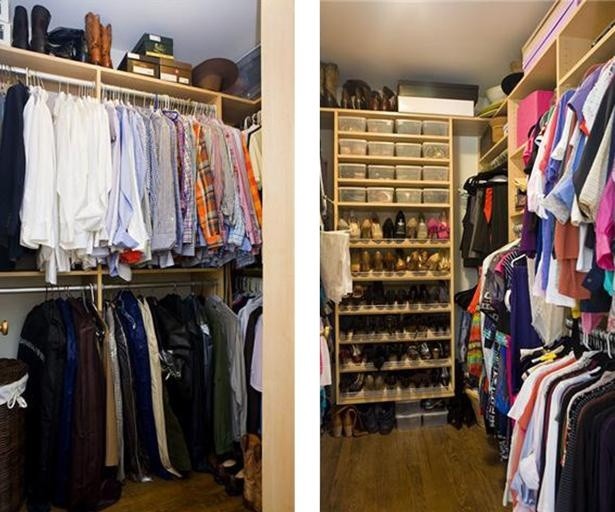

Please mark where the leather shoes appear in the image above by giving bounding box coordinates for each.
[421,400,473,429]
[215,460,243,495]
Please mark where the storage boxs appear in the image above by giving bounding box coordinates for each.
[117,53,160,77]
[397,95,474,117]
[160,57,192,86]
[422,407,448,426]
[337,116,449,204]
[395,403,422,428]
[397,80,479,106]
[131,33,174,58]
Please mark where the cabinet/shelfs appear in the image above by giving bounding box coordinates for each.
[334,108,452,403]
[0,46,261,274]
[478,0,615,244]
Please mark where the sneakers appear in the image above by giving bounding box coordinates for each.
[339,280,449,309]
[339,367,450,398]
[339,343,450,369]
[339,315,450,341]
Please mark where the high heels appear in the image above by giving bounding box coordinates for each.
[338,209,450,243]
[349,248,449,276]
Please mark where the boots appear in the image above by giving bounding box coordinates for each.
[320,61,396,111]
[85,12,100,67]
[330,404,353,437]
[99,24,112,68]
[31,6,52,54]
[360,403,393,435]
[13,3,27,53]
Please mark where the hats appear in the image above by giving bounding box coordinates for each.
[192,57,239,93]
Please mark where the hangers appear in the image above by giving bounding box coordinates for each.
[0,64,217,117]
[512,223,522,238]
[43,278,218,302]
[564,321,615,361]
[234,111,261,130]
[234,271,261,293]
